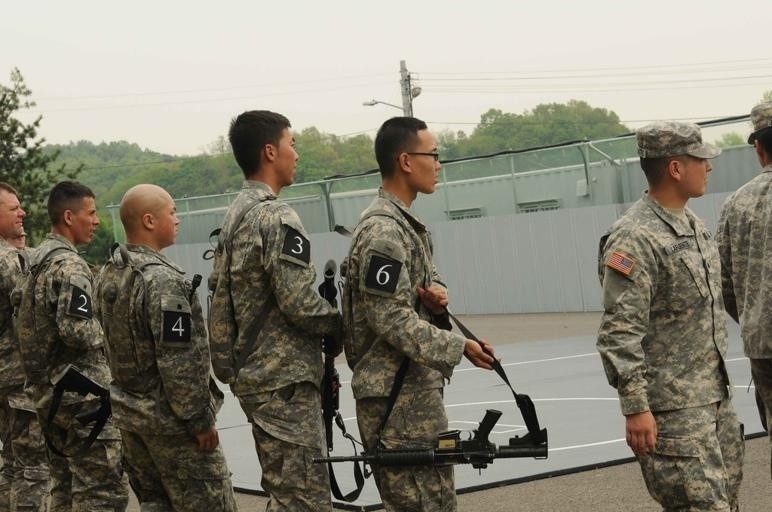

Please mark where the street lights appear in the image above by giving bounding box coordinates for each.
[363,72,421,119]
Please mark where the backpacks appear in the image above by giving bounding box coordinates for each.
[98,242,167,401]
[202,194,282,385]
[342,208,407,371]
[16,248,94,386]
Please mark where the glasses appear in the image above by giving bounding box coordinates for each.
[396,151,439,161]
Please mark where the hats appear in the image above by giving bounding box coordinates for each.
[748,101,772,144]
[636,120,723,159]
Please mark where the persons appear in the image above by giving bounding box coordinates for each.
[214,111,342,512]
[597,120,747,512]
[0,179,52,512]
[343,117,503,512]
[715,99,772,479]
[9,226,35,252]
[92,183,237,512]
[12,180,131,512]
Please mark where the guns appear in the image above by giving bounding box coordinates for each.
[313,394,548,478]
[52,365,112,425]
[318,260,338,453]
